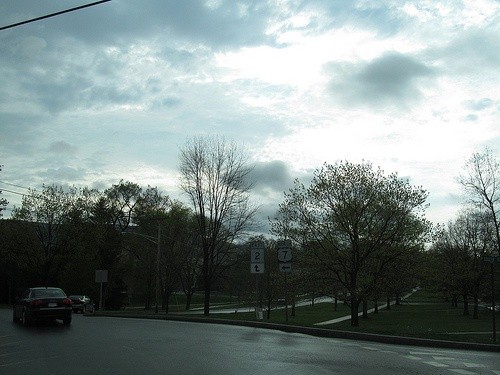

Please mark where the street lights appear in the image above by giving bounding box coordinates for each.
[149,207,162,308]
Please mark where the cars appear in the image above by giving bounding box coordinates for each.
[68,295,95,313]
[12,287,72,328]
